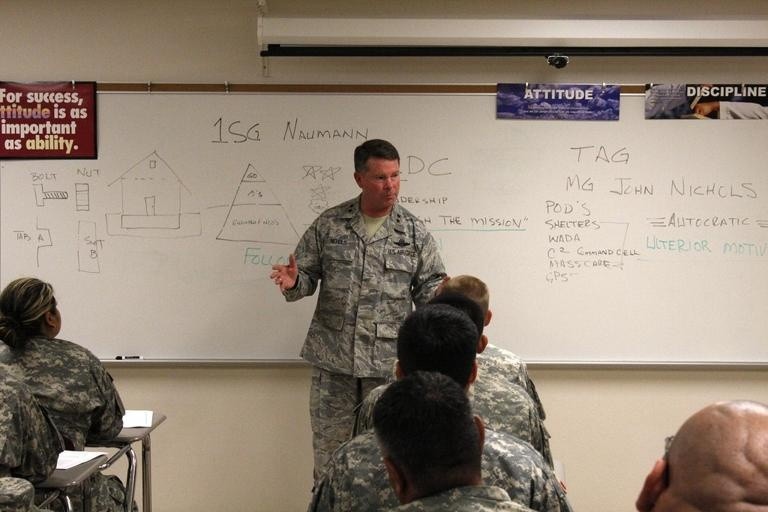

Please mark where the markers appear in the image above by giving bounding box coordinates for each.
[114,356,143,360]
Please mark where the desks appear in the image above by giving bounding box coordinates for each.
[97,411,168,512]
[38,453,108,512]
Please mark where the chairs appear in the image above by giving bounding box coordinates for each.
[38,404,136,512]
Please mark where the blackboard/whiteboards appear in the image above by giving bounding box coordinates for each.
[0,82,768,369]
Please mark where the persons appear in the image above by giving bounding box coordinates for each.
[353,294,554,471]
[0,277,138,512]
[635,398,768,511]
[438,275,547,418]
[371,371,535,512]
[306,304,573,512]
[0,338,71,512]
[270,137,451,493]
[646,85,768,119]
[0,477,45,512]
